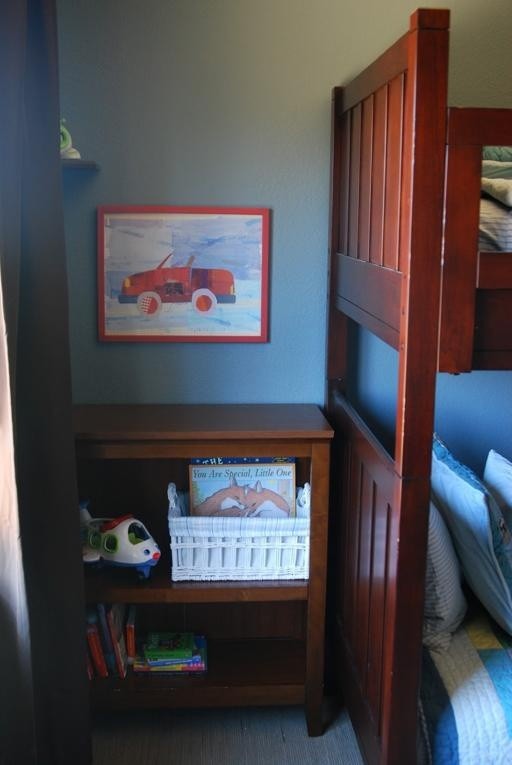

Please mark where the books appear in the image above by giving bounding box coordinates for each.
[83,600,207,682]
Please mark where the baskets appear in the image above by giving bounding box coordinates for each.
[166,481,310,582]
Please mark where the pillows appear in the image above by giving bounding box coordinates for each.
[424,433,511,653]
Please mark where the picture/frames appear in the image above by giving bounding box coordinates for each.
[97,206,271,344]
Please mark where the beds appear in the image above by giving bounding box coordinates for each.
[322,8,512,765]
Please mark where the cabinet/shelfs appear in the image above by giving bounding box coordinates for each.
[70,404,335,738]
